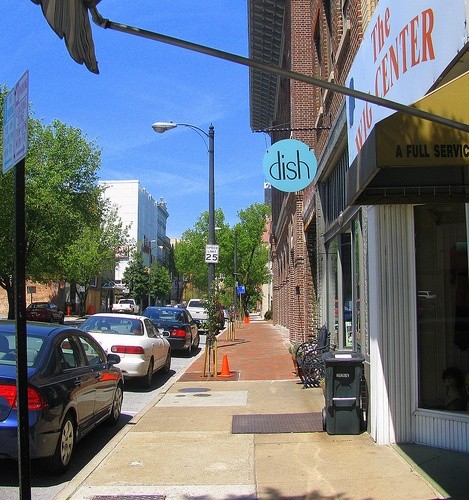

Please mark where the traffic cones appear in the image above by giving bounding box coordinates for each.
[217,353,234,377]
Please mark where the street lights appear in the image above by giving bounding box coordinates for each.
[150,120,218,378]
[214,226,238,323]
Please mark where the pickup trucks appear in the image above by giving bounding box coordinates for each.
[111,298,140,315]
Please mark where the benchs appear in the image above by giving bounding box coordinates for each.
[294,325,330,388]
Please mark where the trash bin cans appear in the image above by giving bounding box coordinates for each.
[322,351,366,434]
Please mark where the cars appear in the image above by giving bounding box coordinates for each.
[25,301,65,325]
[0,317,125,476]
[157,297,240,335]
[129,306,200,358]
[60,311,171,388]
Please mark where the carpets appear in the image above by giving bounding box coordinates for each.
[231,412,325,434]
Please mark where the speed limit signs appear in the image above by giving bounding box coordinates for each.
[204,244,219,264]
